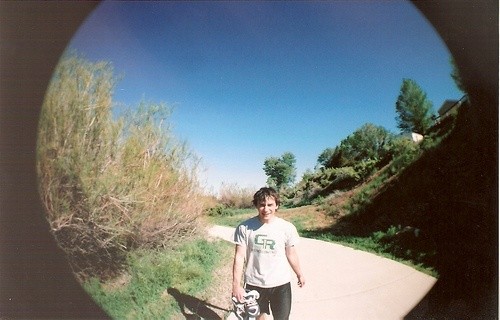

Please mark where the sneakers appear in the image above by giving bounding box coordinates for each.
[231,290,260,320]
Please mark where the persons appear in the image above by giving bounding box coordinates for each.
[232,187,306,320]
